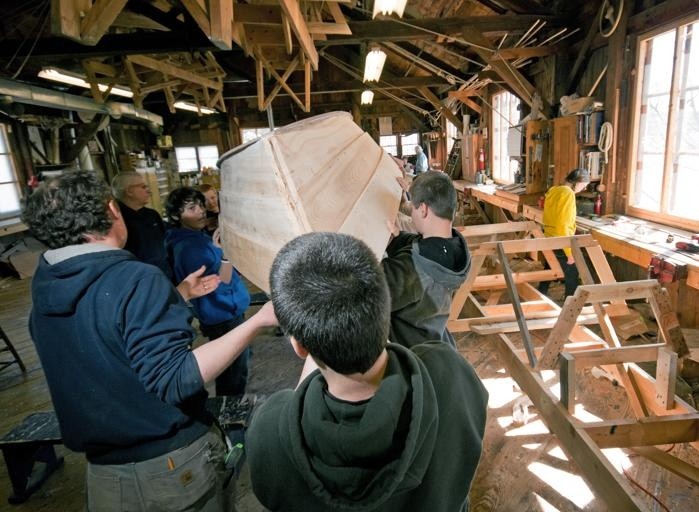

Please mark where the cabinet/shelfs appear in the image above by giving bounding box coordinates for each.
[508,108,604,198]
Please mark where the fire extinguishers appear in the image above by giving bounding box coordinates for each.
[478,146,485,170]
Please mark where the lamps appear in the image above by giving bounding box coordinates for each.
[37,13,137,98]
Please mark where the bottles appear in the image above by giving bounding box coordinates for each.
[595,194,602,215]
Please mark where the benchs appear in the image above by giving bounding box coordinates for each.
[1,393,258,507]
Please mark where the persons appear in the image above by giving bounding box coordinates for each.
[379,171,473,348]
[536,167,591,301]
[18,171,284,512]
[242,232,488,511]
[112,166,253,397]
[387,145,428,201]
